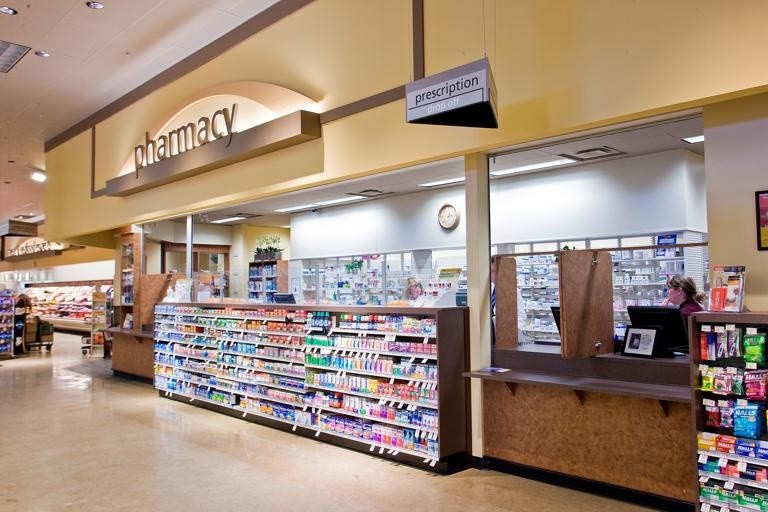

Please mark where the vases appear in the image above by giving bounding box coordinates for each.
[254,253,281,260]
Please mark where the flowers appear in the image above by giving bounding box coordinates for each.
[253,233,284,253]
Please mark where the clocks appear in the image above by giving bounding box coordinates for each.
[437,203,460,232]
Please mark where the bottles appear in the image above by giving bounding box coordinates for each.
[125,287,130,303]
[167,285,173,301]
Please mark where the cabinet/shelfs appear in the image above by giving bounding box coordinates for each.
[90,291,111,355]
[303,252,467,303]
[249,260,288,303]
[688,311,768,512]
[0,295,28,358]
[153,303,468,478]
[120,243,134,304]
[25,280,113,333]
[496,232,703,343]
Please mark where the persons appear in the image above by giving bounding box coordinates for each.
[404,277,423,299]
[660,274,705,319]
[490,254,526,342]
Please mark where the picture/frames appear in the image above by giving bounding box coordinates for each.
[622,324,661,357]
[755,189,768,251]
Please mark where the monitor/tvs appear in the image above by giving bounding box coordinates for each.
[550,306,561,333]
[273,293,296,304]
[626,306,688,358]
[456,289,467,306]
